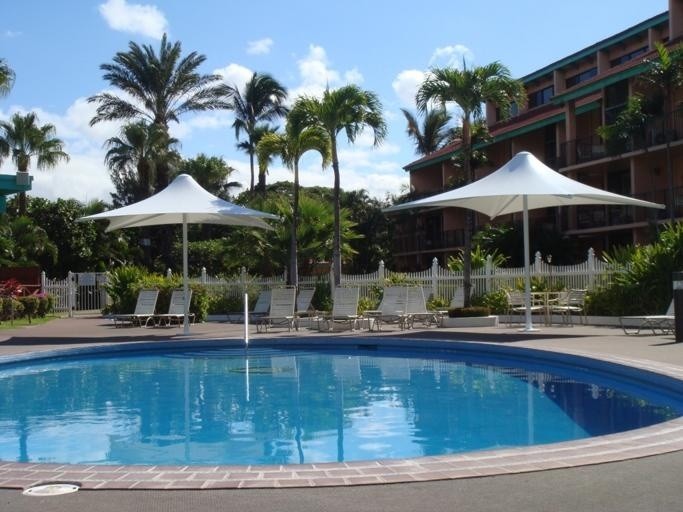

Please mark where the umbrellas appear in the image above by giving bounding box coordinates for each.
[381,151,666,331]
[76,172,282,332]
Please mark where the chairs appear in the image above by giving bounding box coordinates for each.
[111,289,196,330]
[617,297,676,337]
[225,282,477,334]
[501,286,588,328]
